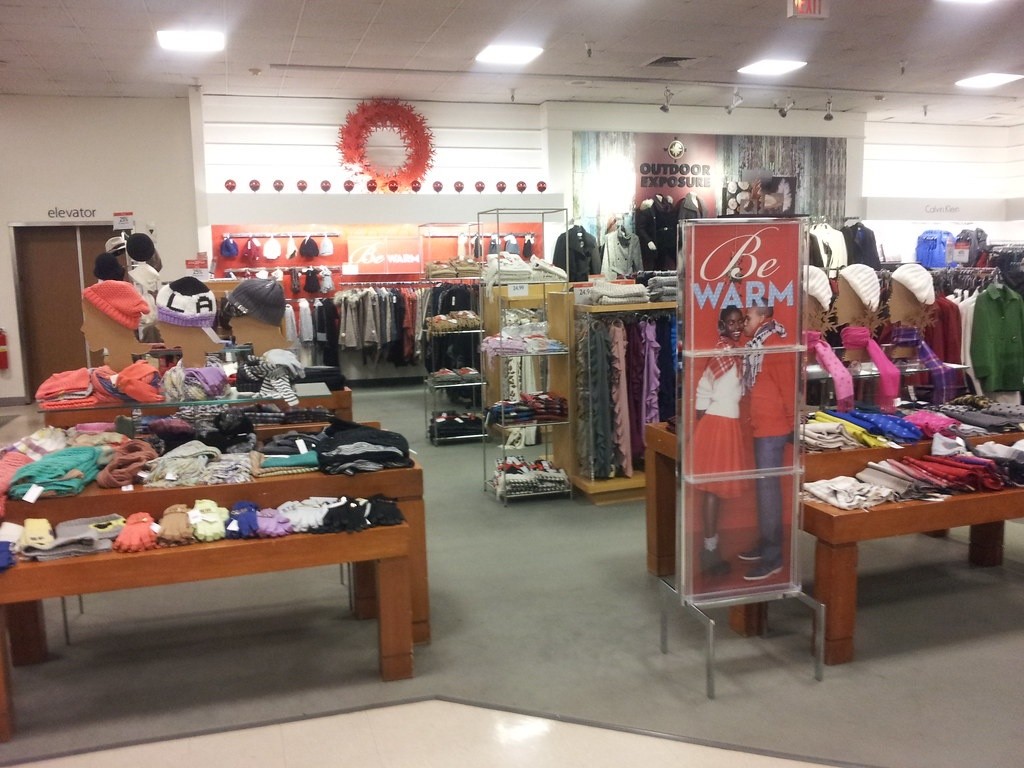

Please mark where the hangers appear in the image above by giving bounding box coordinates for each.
[574,215,583,240]
[808,213,1024,297]
[621,215,625,235]
[345,279,478,297]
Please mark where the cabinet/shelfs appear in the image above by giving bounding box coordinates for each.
[419,207,677,507]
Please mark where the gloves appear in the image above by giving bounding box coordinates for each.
[113,495,406,551]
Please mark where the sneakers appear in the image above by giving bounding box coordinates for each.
[744,559,783,581]
[738,545,762,559]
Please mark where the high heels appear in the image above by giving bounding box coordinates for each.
[699,547,732,577]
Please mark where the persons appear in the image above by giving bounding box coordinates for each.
[690,297,794,580]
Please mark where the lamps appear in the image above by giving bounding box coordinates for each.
[823,99,833,120]
[779,94,796,117]
[660,84,674,112]
[725,86,744,115]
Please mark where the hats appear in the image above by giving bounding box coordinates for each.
[33,366,97,411]
[93,233,162,324]
[82,280,150,330]
[840,264,881,312]
[802,264,833,313]
[223,278,285,326]
[891,264,936,305]
[219,236,335,260]
[155,277,216,328]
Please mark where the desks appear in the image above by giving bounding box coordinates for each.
[643,360,1024,663]
[0,345,432,743]
